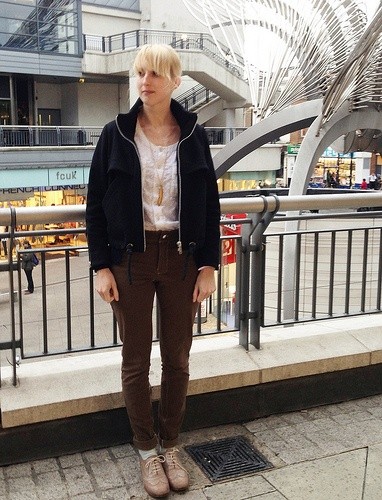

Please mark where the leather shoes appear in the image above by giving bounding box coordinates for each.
[139,454,170,498]
[158,448,189,491]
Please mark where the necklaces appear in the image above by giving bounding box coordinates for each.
[141,114,172,206]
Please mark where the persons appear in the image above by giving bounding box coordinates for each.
[1,228,8,255]
[16,240,35,295]
[85,42,222,498]
[369,172,377,190]
[13,226,26,247]
[360,178,368,190]
[327,169,338,188]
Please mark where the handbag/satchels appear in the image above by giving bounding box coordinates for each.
[32,254,39,265]
